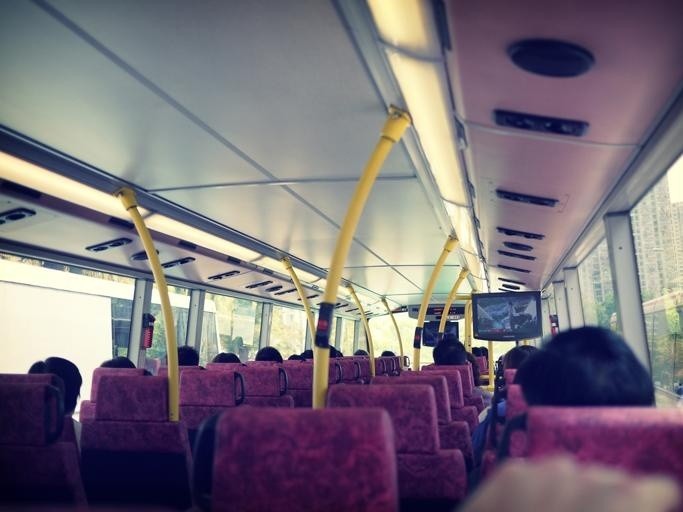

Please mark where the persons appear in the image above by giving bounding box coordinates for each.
[513,325,654,405]
[433,333,540,466]
[354,349,369,355]
[100,356,136,368]
[211,352,241,363]
[381,351,395,356]
[28,357,83,459]
[178,344,200,366]
[288,344,343,361]
[255,346,283,363]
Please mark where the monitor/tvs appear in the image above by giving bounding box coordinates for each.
[472,291,543,341]
[422,321,460,347]
